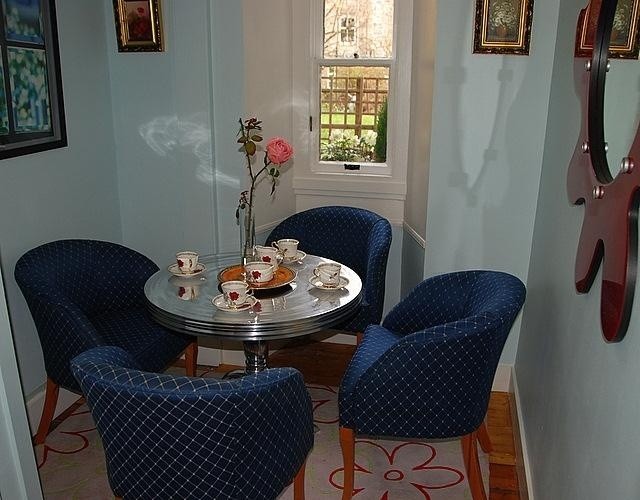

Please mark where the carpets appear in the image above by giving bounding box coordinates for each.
[33,366,490,499]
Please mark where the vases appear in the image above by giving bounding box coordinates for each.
[239,205,261,270]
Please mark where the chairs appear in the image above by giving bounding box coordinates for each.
[68,346,315,499]
[264,205,394,347]
[337,270,527,499]
[13,239,199,446]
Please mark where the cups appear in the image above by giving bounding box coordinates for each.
[167,238,349,314]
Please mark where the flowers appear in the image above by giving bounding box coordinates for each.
[234,116,295,258]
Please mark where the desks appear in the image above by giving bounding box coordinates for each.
[143,246,364,376]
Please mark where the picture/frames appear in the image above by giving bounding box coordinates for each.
[112,0,166,53]
[574,0,640,60]
[471,0,535,56]
